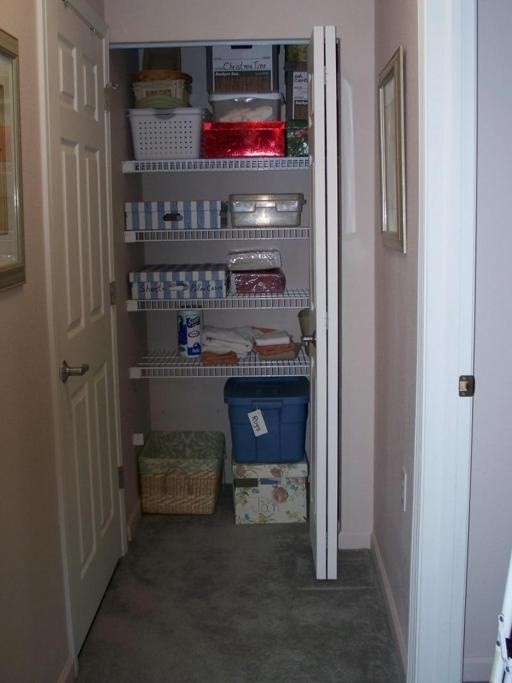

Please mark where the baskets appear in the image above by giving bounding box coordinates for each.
[129,77,207,158]
[139,428,225,513]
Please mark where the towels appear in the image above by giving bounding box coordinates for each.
[203,325,303,366]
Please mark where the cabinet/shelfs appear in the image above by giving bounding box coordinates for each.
[119,156,315,379]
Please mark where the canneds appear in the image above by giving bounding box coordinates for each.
[178,306,203,358]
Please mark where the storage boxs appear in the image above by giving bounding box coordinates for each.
[223,376,309,529]
[128,263,227,299]
[202,43,309,157]
[125,192,302,229]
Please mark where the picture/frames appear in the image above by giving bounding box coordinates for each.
[0,28,26,292]
[376,46,407,253]
